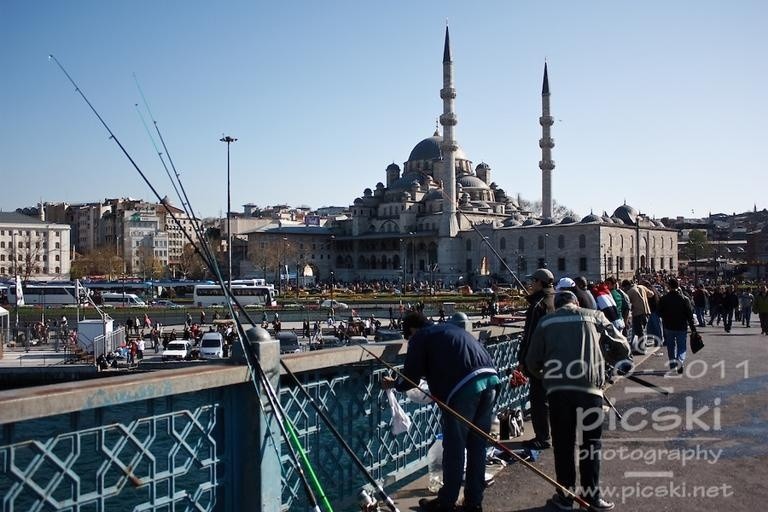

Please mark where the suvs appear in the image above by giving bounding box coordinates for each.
[274,329,300,355]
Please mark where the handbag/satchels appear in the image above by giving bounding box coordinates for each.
[689,332,703,353]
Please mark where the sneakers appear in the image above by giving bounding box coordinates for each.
[419,498,483,512]
[551,493,573,509]
[522,437,552,448]
[580,499,614,509]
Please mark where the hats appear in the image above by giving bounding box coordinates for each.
[556,275,576,290]
[526,268,554,285]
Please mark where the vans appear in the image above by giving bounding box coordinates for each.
[197,332,226,360]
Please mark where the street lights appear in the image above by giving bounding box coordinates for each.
[600,243,612,280]
[514,249,520,287]
[688,239,703,285]
[114,232,121,256]
[401,232,413,297]
[544,233,548,268]
[296,260,300,292]
[475,236,489,293]
[283,237,288,289]
[329,272,334,319]
[706,249,722,287]
[14,233,18,311]
[219,135,238,318]
[329,235,335,286]
[150,245,155,299]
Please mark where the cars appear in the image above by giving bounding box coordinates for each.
[374,325,403,342]
[514,309,527,320]
[162,339,195,362]
[473,282,518,294]
[309,322,348,351]
[0,279,284,308]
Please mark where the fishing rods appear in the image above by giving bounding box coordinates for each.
[340,316,524,329]
[417,166,623,421]
[311,310,600,512]
[606,361,677,396]
[49,53,399,512]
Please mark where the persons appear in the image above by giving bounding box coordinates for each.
[7,278,499,367]
[378,309,502,511]
[516,266,768,512]
